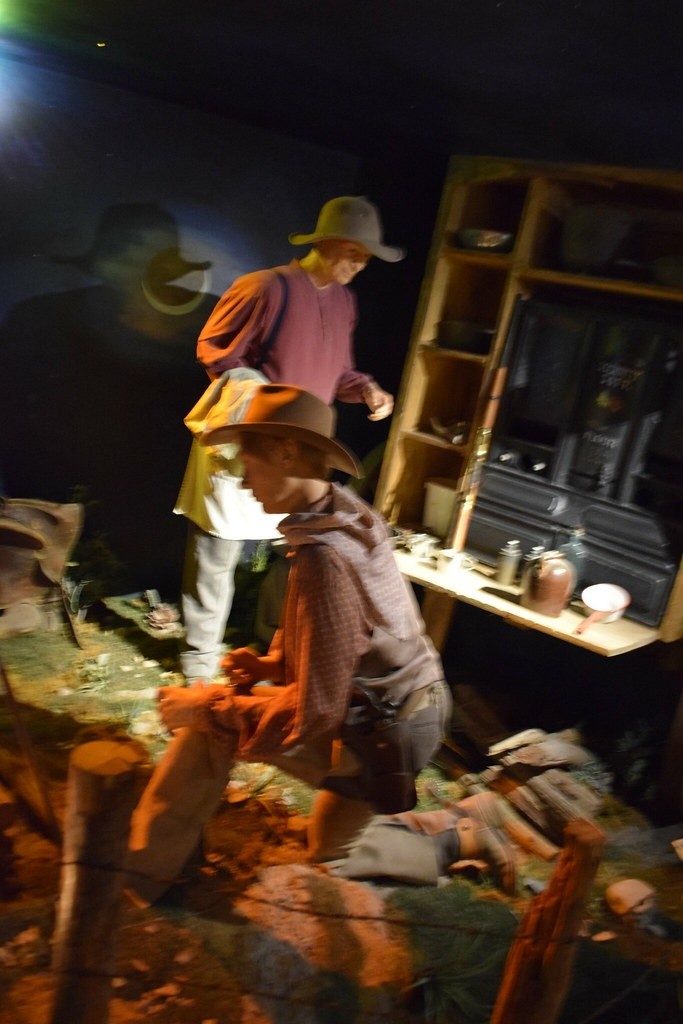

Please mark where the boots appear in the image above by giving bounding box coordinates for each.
[112,864,175,898]
[419,792,524,897]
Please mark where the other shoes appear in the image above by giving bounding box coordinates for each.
[178,652,221,692]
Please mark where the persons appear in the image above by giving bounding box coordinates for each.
[122,384,517,908]
[178,196,403,687]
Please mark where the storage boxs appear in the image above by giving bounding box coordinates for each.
[421,478,459,537]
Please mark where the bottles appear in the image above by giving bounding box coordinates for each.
[558,529,588,608]
[497,540,519,585]
[522,547,540,589]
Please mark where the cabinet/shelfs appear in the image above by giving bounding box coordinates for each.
[373,157,683,642]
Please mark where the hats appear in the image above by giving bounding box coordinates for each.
[198,382,362,478]
[290,195,403,266]
